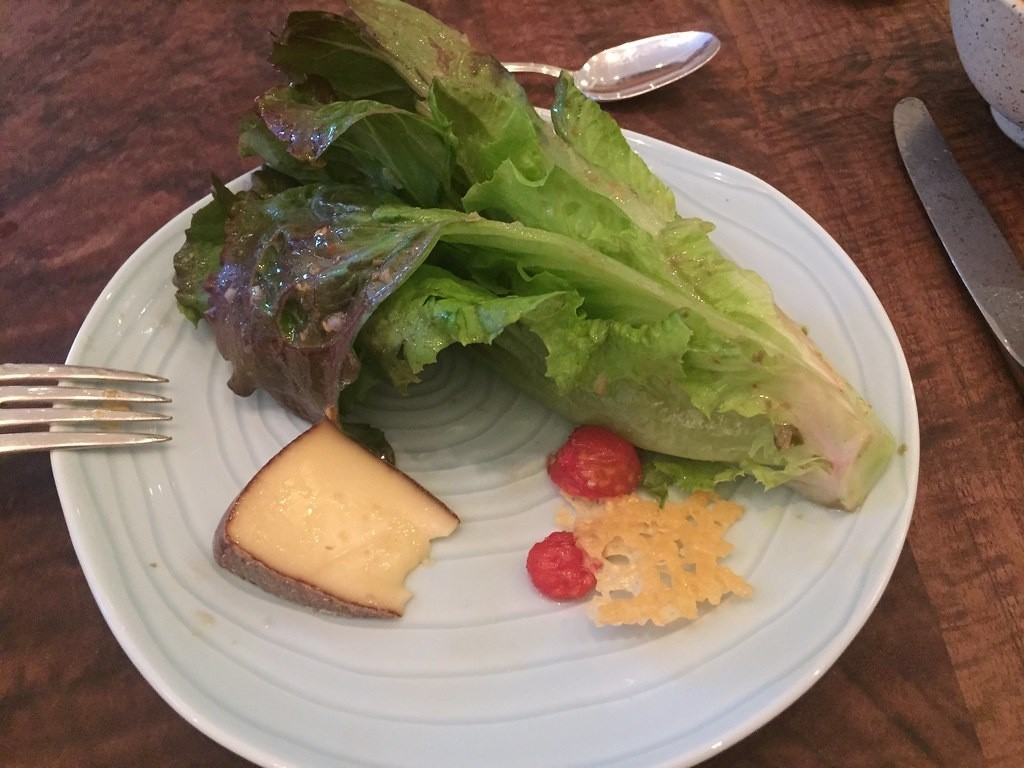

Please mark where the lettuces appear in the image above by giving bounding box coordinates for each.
[172,2,896,510]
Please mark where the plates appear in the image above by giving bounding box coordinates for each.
[48,106,920,768]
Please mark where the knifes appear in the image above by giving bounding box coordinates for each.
[895,96,1024,368]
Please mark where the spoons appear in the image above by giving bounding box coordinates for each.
[502,30,720,101]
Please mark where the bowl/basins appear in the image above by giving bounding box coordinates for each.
[947,1,1024,151]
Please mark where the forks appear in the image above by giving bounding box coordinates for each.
[0,363,172,454]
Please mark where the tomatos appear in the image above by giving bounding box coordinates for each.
[527,530,604,601]
[547,423,641,499]
